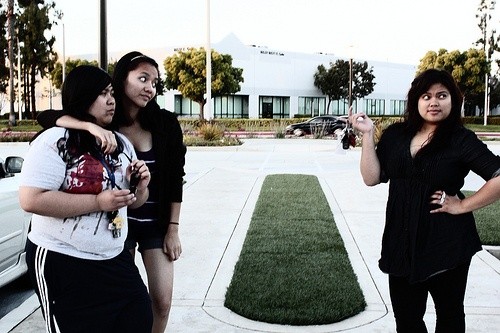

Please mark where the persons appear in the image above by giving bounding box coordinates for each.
[19,65,153,333]
[36,51,187,333]
[348,69,500,333]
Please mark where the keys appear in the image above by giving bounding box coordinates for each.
[340,127,356,149]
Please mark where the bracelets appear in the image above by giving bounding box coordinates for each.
[169,222,179,225]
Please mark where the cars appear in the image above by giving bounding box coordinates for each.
[286,116,348,136]
[0,142,32,288]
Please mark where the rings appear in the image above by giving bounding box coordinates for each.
[440,191,446,204]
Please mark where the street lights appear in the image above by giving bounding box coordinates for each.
[347,45,356,131]
[60,8,68,85]
